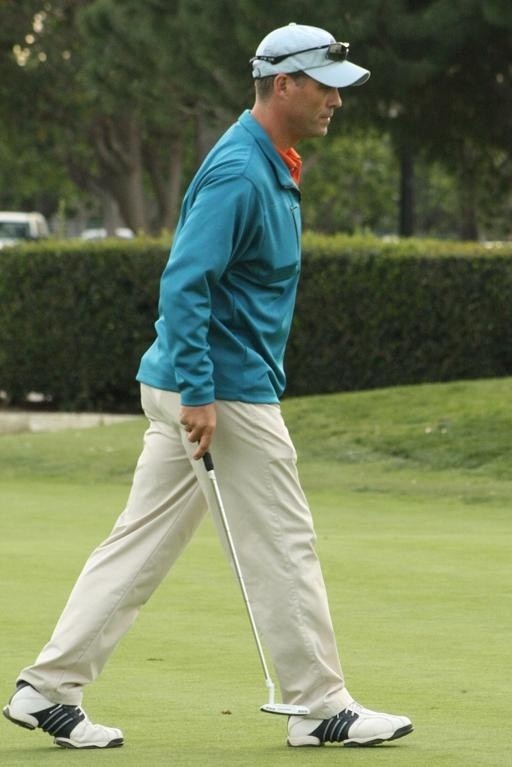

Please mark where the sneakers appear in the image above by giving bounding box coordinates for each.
[287,704,414,747]
[3,681,125,749]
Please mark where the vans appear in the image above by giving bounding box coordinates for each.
[0,209,50,251]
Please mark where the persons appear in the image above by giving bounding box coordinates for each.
[3,22,414,749]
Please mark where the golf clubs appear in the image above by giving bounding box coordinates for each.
[203,448,311,717]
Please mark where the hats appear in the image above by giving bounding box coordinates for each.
[252,22,371,88]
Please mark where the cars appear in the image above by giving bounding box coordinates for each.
[82,226,136,242]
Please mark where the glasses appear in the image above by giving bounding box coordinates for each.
[271,41,351,65]
